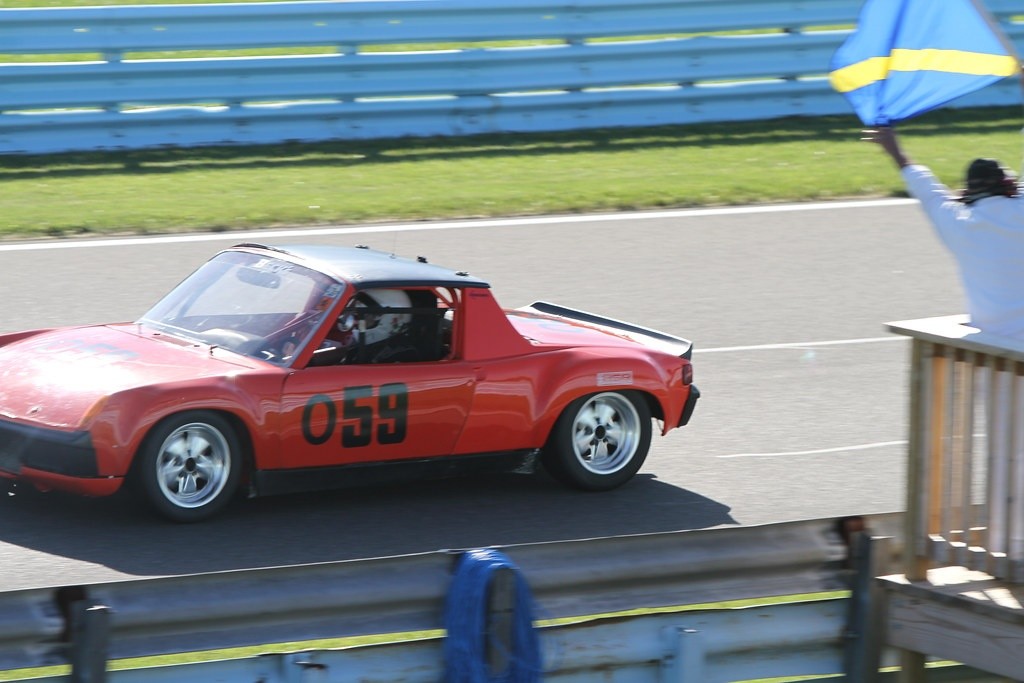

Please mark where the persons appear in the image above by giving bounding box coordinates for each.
[281,289,428,366]
[864,121,1024,583]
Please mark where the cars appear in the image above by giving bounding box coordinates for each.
[0,243,703,521]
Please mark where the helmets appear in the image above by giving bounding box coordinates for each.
[337,288,414,344]
